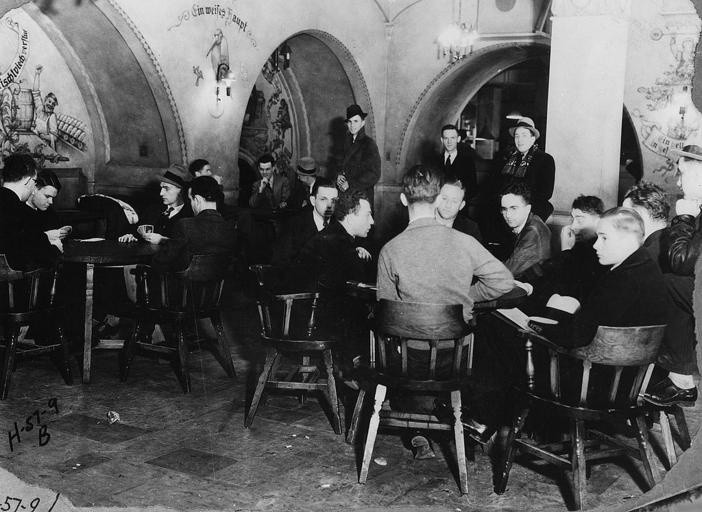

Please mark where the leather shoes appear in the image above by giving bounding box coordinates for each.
[411,435,436,460]
[90,317,117,346]
[435,398,496,445]
[644,378,698,406]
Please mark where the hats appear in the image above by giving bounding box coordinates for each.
[345,104,368,120]
[156,164,192,189]
[509,117,540,139]
[296,158,318,175]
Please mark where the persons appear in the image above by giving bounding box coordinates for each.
[329,104,381,217]
[433,124,473,184]
[623,184,672,271]
[95,164,191,338]
[189,159,224,214]
[290,157,328,206]
[434,178,487,251]
[240,151,290,216]
[1,154,67,348]
[502,117,554,223]
[309,189,375,390]
[643,145,700,408]
[556,196,603,292]
[26,170,72,242]
[377,167,514,459]
[290,180,337,259]
[520,208,662,449]
[140,175,231,346]
[501,185,553,286]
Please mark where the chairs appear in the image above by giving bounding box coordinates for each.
[245,263,342,433]
[118,253,236,392]
[498,325,668,512]
[348,299,475,493]
[0,254,77,400]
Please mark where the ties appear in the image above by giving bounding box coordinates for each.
[446,155,451,166]
[165,207,174,217]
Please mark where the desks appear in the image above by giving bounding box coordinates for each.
[56,236,178,384]
[347,280,529,420]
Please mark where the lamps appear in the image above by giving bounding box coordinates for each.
[209,63,230,119]
[433,19,480,65]
